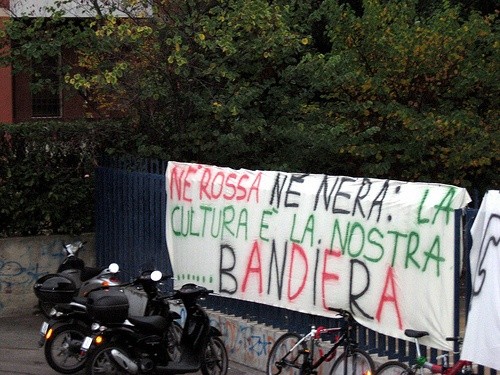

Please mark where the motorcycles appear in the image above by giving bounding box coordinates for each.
[33,270,183,375]
[78,284,229,375]
[38,240,92,319]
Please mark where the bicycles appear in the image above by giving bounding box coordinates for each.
[375,329,472,375]
[266,306,375,375]
